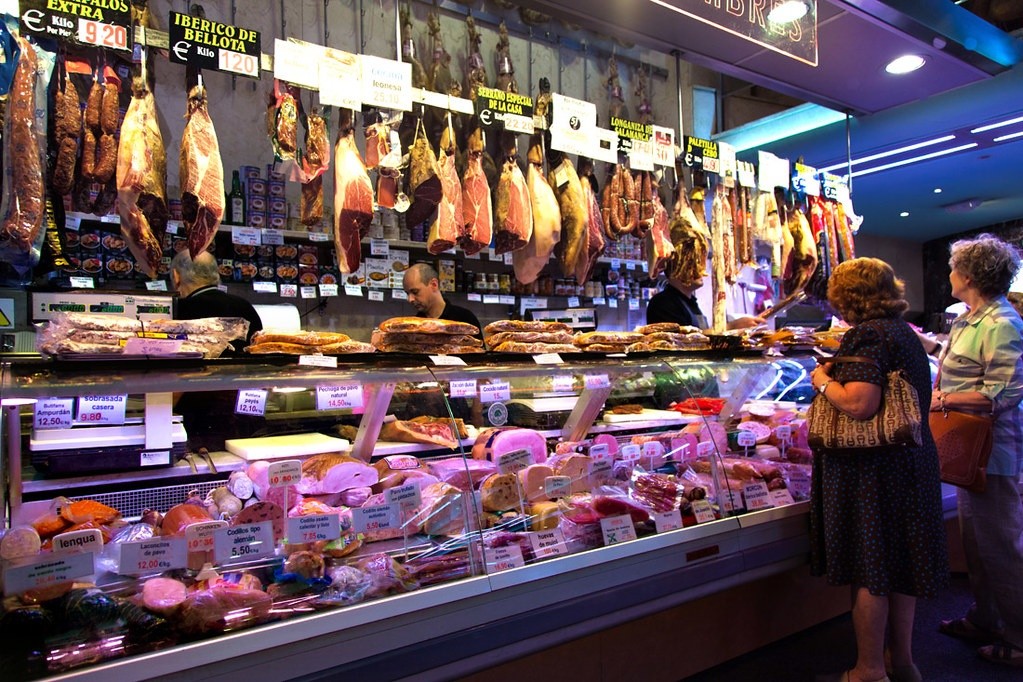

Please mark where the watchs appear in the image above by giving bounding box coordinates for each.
[820,378,835,396]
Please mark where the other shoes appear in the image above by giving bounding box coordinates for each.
[883,654,923,682]
[815,669,890,682]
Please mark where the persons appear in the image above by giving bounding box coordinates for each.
[782,255,851,331]
[644,254,767,414]
[168,249,263,460]
[804,257,941,682]
[911,235,1023,670]
[401,263,491,425]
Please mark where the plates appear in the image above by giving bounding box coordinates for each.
[41,346,770,371]
[217,243,337,284]
[62,233,215,274]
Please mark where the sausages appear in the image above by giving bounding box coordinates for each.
[600,165,658,240]
[47,72,139,226]
[732,186,752,263]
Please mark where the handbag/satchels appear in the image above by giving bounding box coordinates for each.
[928,409,994,488]
[808,356,924,448]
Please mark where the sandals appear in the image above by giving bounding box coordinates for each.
[977,644,1023,666]
[940,615,1001,641]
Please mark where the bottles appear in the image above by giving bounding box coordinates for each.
[228,170,245,226]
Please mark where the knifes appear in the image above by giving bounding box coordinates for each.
[596,420,620,427]
[194,436,217,474]
[181,442,198,474]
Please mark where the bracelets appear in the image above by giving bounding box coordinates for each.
[939,392,950,410]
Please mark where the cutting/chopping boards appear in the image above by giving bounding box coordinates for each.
[603,407,681,422]
[224,431,350,460]
